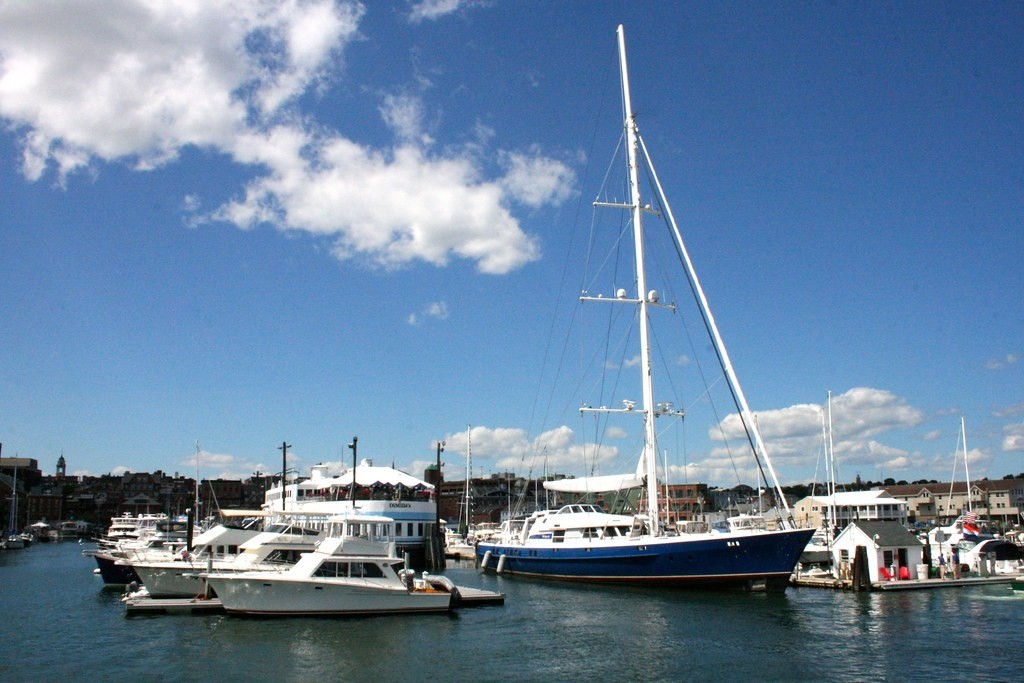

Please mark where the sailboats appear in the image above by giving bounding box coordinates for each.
[475,23,818,595]
[927,416,996,545]
[676,415,767,535]
[799,391,843,564]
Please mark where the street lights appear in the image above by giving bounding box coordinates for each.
[277,442,292,511]
[348,437,358,508]
[936,509,944,555]
[436,440,446,529]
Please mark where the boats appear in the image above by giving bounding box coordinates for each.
[114,488,265,598]
[467,523,500,540]
[47,521,88,539]
[188,522,328,573]
[20,533,35,547]
[956,539,1023,574]
[439,518,475,557]
[82,513,205,584]
[6,535,24,549]
[183,516,463,619]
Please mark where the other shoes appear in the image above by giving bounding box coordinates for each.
[941,577,961,581]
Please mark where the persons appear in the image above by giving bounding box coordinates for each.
[939,553,946,579]
[951,550,960,579]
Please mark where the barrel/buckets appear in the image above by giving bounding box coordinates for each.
[916,564,928,579]
